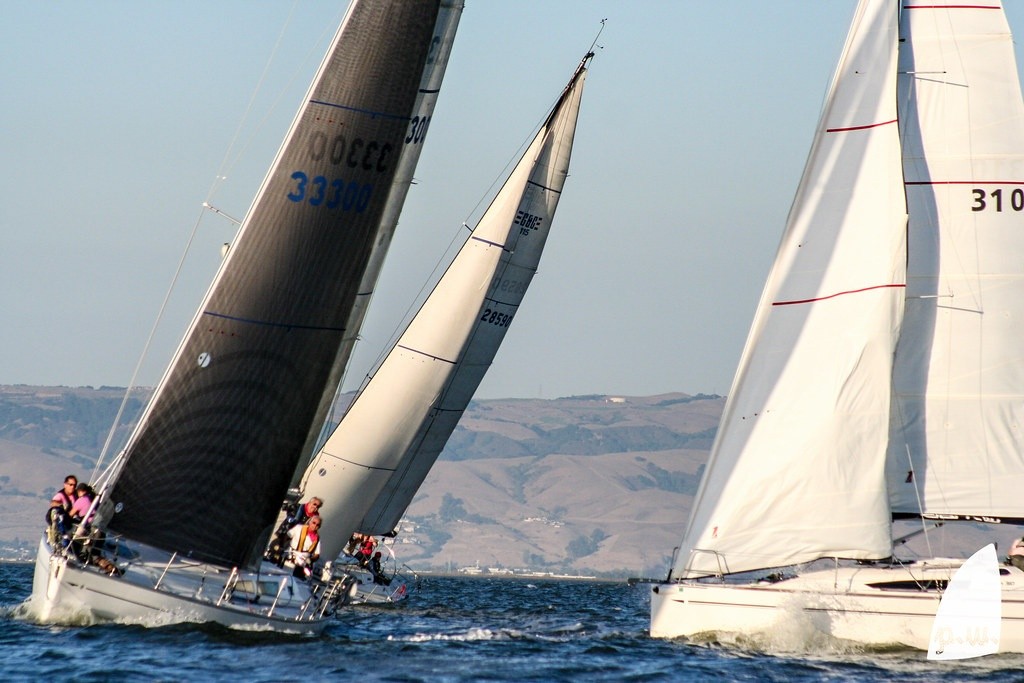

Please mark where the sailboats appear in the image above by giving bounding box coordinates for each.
[28,0,606,631]
[648,1,1024,662]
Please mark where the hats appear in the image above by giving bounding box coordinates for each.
[375,552,381,557]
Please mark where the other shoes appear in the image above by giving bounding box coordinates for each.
[109,567,115,577]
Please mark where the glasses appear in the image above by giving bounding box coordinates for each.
[313,502,320,509]
[64,482,76,487]
[311,521,320,526]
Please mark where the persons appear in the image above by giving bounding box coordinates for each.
[273,498,323,577]
[47,475,108,563]
[346,533,382,583]
